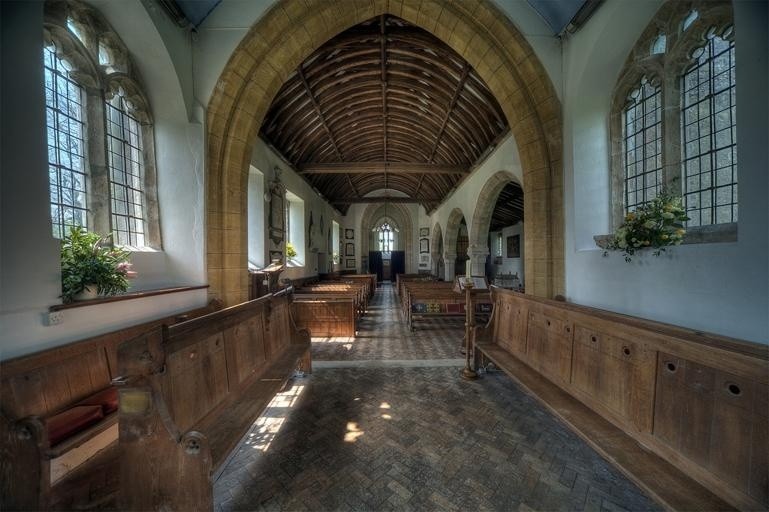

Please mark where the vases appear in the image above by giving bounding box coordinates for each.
[70,277,100,301]
[287,259,292,266]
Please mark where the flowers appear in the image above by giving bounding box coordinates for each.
[56,228,139,301]
[600,186,692,263]
[285,244,297,258]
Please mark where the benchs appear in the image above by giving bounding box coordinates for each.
[0,297,313,512]
[286,272,378,338]
[395,272,491,336]
[467,290,768,512]
